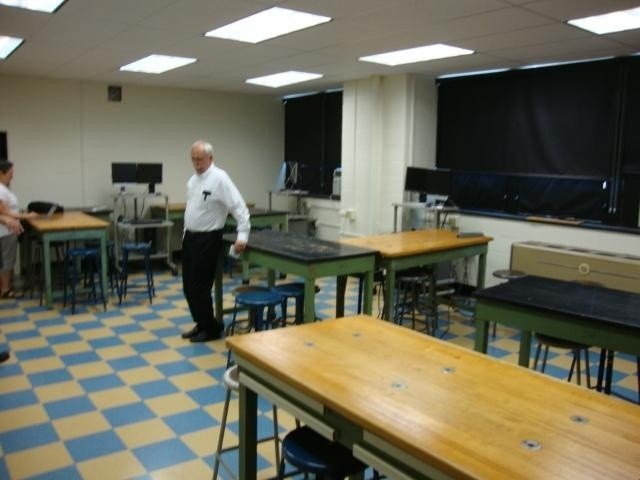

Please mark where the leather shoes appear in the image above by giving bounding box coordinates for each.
[182,325,201,338]
[190,329,221,342]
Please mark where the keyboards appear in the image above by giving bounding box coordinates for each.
[131,218,162,224]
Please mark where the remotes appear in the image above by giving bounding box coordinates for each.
[459,232,484,237]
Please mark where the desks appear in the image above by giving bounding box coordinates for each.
[17,203,290,312]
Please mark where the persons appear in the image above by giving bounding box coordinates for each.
[180,140,252,342]
[0,159,40,300]
[0,215,25,237]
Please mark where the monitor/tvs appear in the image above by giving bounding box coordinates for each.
[137,163,162,193]
[112,164,137,192]
[405,165,452,202]
